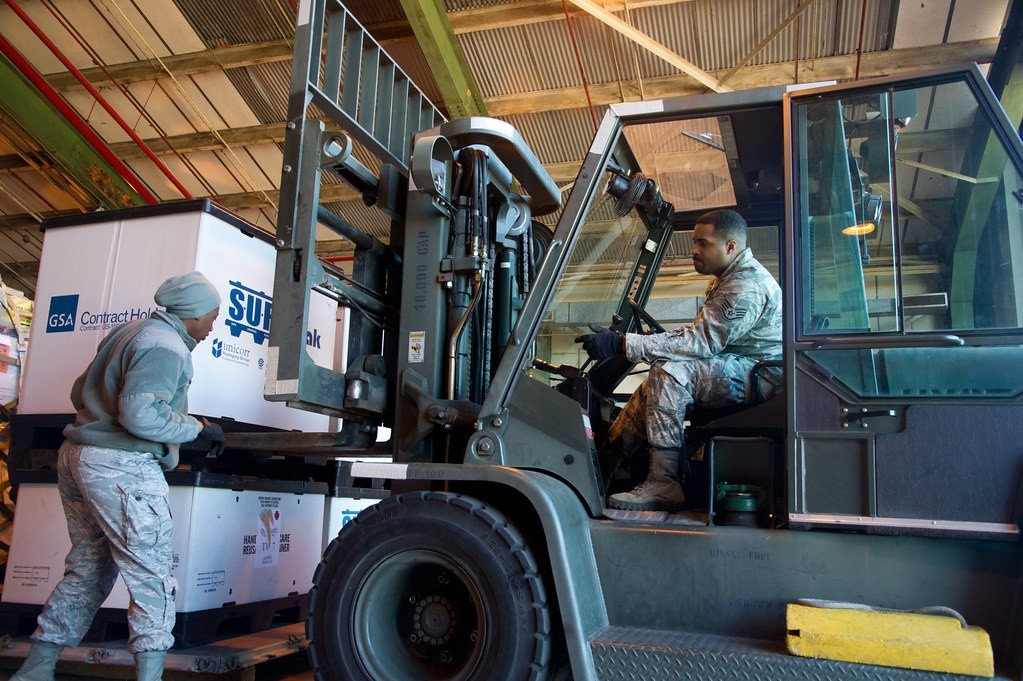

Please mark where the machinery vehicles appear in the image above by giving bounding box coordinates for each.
[189,0,1023,681]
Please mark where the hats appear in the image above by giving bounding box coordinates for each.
[154,271,222,320]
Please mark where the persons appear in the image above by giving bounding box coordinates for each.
[574,209,784,513]
[9,271,227,681]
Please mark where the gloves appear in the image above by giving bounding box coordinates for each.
[199,419,225,457]
[574,323,625,362]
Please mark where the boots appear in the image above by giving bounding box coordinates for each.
[9,641,64,681]
[608,449,686,512]
[133,650,167,681]
